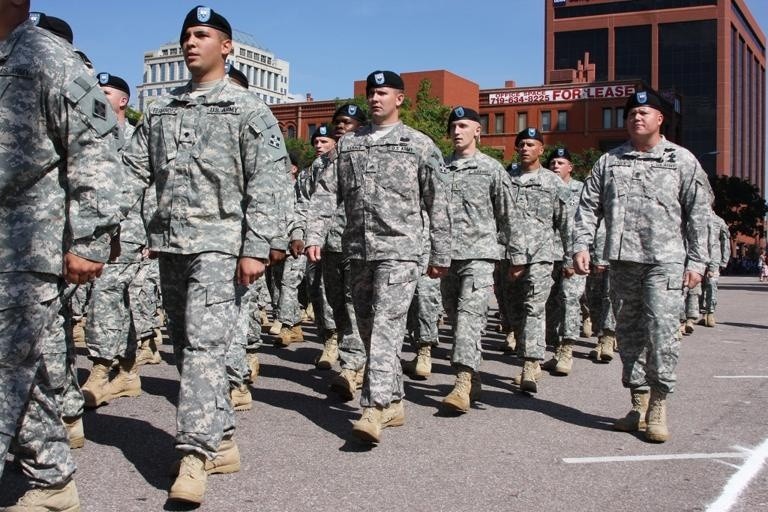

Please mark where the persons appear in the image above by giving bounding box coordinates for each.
[758,249,768,282]
[681,211,731,334]
[569,85,715,443]
[1,0,293,510]
[265,69,617,440]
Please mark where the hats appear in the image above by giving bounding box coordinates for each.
[29,12,73,43]
[515,126,543,147]
[447,105,480,129]
[310,125,334,147]
[224,62,249,89]
[331,103,367,126]
[181,4,232,37]
[505,163,521,176]
[365,70,404,89]
[547,148,572,167]
[71,50,94,70]
[622,90,663,120]
[95,72,130,96]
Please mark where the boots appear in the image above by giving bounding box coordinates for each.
[415,345,432,378]
[104,353,141,399]
[79,358,110,408]
[644,388,668,444]
[614,391,652,432]
[552,338,575,373]
[442,363,472,411]
[316,328,339,369]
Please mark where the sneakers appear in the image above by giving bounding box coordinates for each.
[582,316,593,337]
[760,279,768,281]
[470,374,482,401]
[514,360,542,393]
[680,315,695,336]
[701,313,715,327]
[505,331,518,355]
[589,336,618,361]
[231,384,252,411]
[380,398,405,429]
[352,405,380,444]
[0,479,80,512]
[135,326,163,364]
[245,352,259,380]
[328,363,367,401]
[71,317,87,348]
[258,299,315,346]
[168,452,206,501]
[65,414,86,448]
[205,434,240,476]
